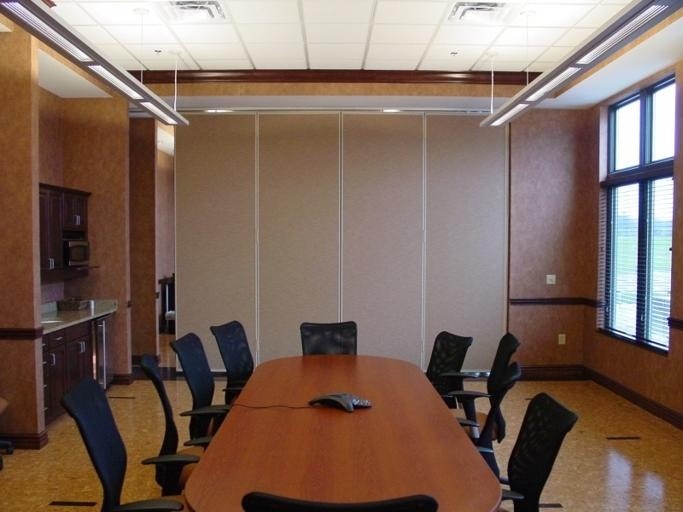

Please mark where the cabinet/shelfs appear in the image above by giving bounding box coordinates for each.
[39,182,93,285]
[43,328,66,425]
[92,311,118,391]
[66,318,92,425]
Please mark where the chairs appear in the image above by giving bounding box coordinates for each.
[501,393,578,512]
[445,363,521,468]
[210,320,255,404]
[423,332,472,405]
[137,354,211,497]
[433,334,519,437]
[171,332,242,441]
[239,492,439,512]
[58,382,198,512]
[298,320,358,357]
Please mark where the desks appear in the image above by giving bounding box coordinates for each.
[184,355,502,512]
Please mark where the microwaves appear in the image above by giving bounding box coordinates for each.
[64,237,90,267]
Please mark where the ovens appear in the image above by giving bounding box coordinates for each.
[91,315,114,392]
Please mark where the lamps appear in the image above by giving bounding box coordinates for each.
[476,1,680,129]
[1,0,189,126]
